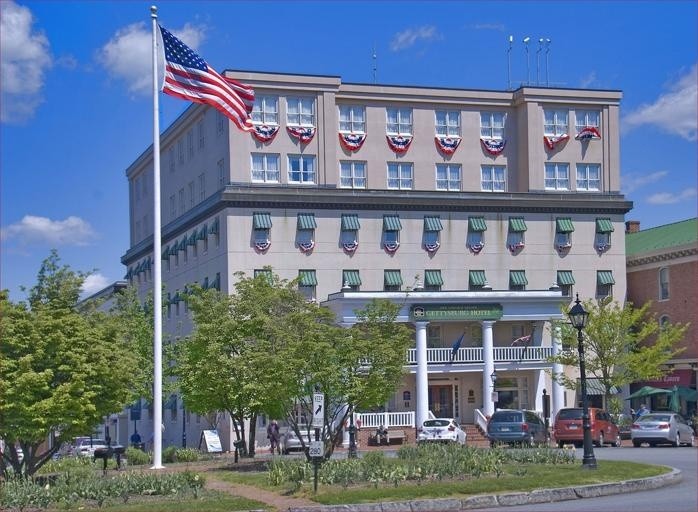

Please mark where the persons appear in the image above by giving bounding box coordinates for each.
[266,418,282,455]
[130,429,142,450]
[629,407,636,421]
[689,409,697,438]
[634,403,650,418]
[375,424,390,446]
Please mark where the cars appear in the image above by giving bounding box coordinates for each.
[554,407,622,448]
[67,436,125,462]
[630,411,695,448]
[415,418,468,449]
[485,410,550,447]
[281,423,323,455]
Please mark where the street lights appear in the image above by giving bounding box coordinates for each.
[567,294,599,470]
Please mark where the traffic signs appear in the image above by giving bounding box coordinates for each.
[312,391,325,430]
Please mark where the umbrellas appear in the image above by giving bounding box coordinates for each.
[667,384,683,415]
[678,385,697,404]
[623,385,673,406]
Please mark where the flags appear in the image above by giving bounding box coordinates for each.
[511,333,532,347]
[155,21,259,135]
[451,333,464,354]
